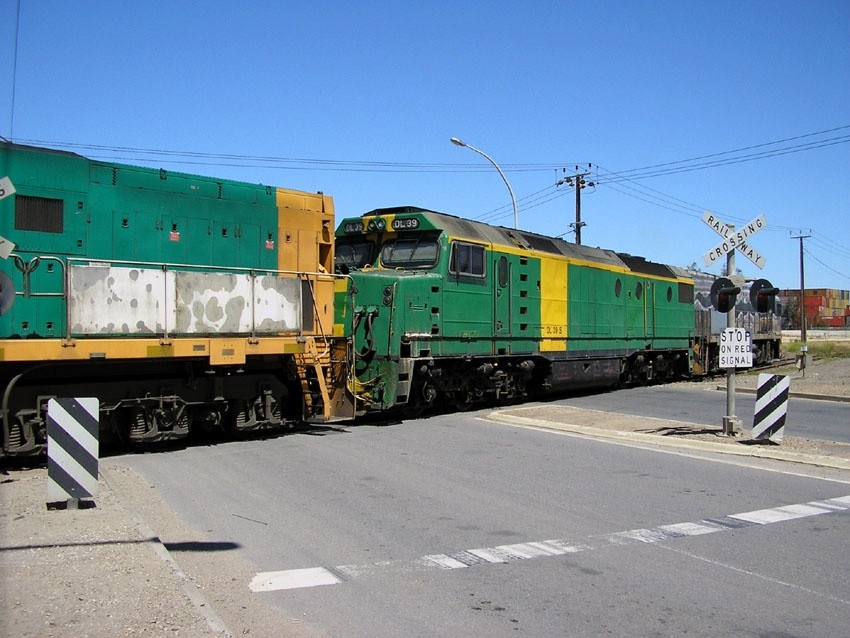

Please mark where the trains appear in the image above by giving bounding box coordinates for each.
[0,138,784,468]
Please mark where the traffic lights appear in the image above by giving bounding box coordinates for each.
[709,276,740,314]
[748,279,778,313]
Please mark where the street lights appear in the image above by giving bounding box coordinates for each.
[449,137,531,235]
